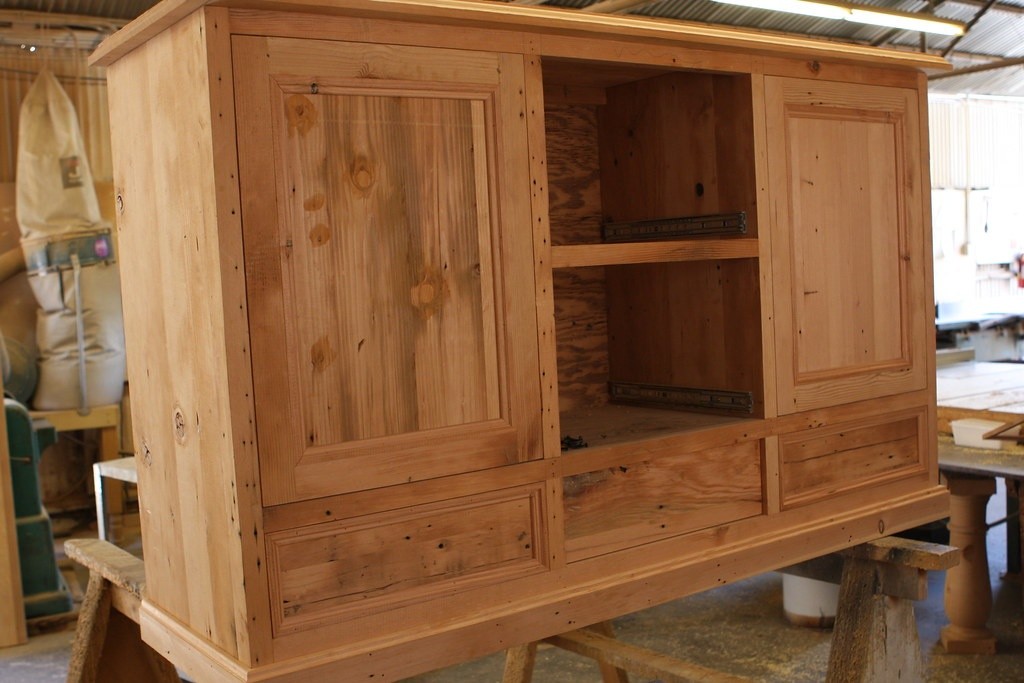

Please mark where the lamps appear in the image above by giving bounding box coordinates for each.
[715,0,965,37]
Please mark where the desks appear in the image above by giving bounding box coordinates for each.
[29,405,125,548]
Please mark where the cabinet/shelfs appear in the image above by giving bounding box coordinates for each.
[84,0,954,683]
